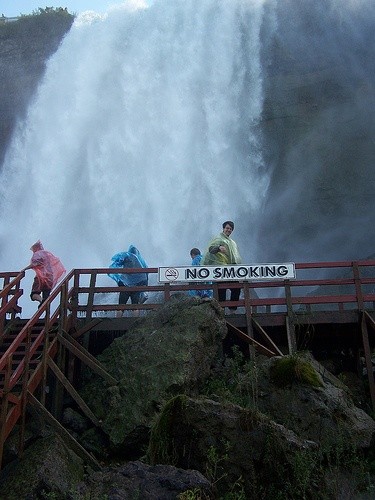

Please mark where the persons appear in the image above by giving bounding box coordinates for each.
[202,219,244,314]
[19,239,66,318]
[108,245,148,317]
[189,247,213,298]
